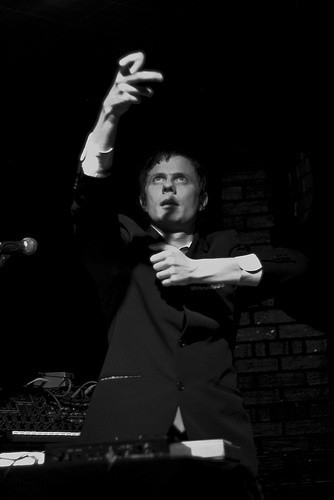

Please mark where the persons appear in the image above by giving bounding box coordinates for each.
[70,52,310,469]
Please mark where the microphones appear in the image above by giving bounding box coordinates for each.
[0,237,38,255]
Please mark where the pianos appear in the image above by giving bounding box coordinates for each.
[0,436,242,470]
